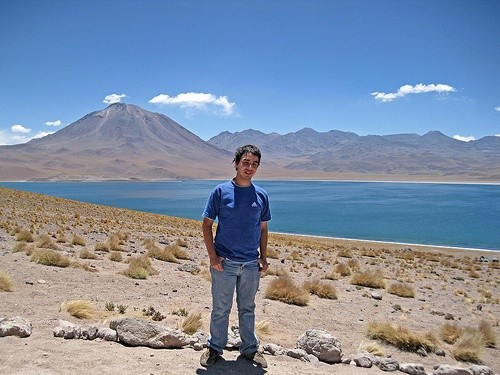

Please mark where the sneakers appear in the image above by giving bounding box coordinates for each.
[246,351,267,368]
[200,346,218,366]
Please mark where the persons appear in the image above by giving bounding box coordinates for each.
[199,145,271,368]
